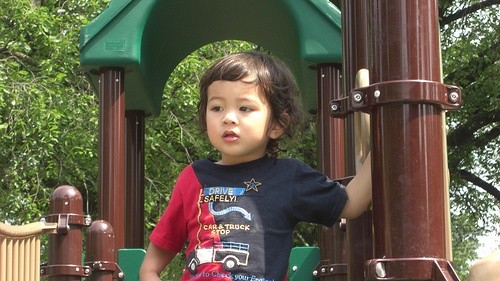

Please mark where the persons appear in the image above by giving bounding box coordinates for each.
[139,51,372,281]
